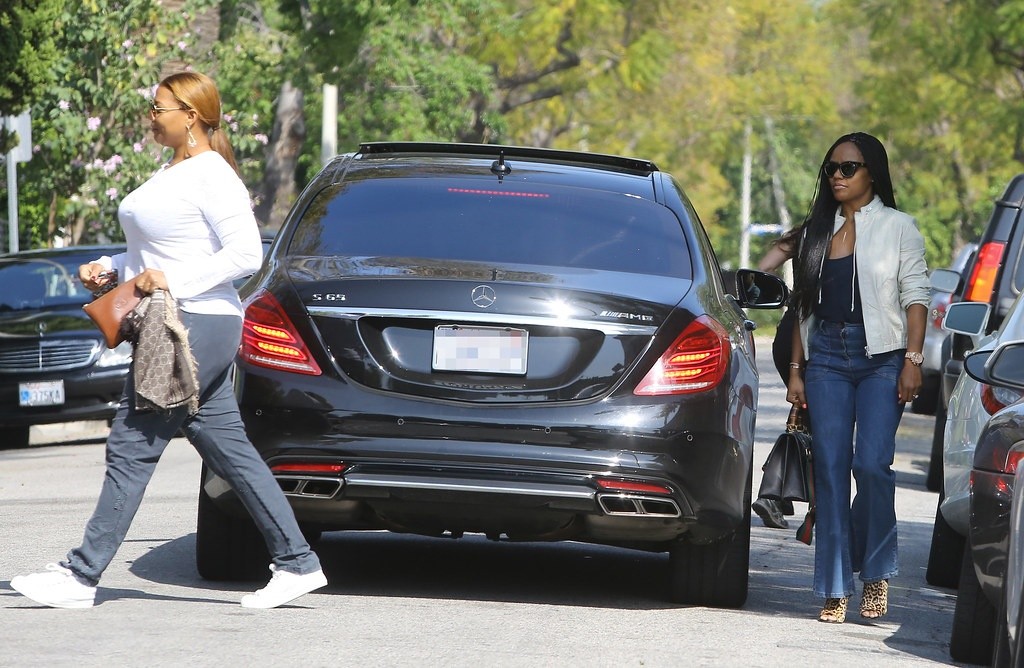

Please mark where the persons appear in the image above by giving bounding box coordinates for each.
[10,71,330,610]
[742,217,821,529]
[750,131,932,623]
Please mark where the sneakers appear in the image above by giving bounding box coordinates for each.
[9,562,97,609]
[241,563,327,609]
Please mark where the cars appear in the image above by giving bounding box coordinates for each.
[0,229,278,449]
[912,171,1024,668]
[197,143,789,608]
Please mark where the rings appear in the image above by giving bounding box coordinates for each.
[912,394,920,399]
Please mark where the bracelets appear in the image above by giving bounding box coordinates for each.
[789,362,804,369]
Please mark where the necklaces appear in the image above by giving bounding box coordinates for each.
[842,229,847,242]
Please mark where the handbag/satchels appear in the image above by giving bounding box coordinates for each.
[82,274,148,349]
[759,399,814,546]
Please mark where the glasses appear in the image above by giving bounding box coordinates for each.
[149,100,190,119]
[823,162,868,179]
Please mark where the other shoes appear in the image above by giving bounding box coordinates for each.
[859,578,888,618]
[819,597,849,622]
[752,494,789,529]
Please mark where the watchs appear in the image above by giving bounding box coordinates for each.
[904,351,925,366]
